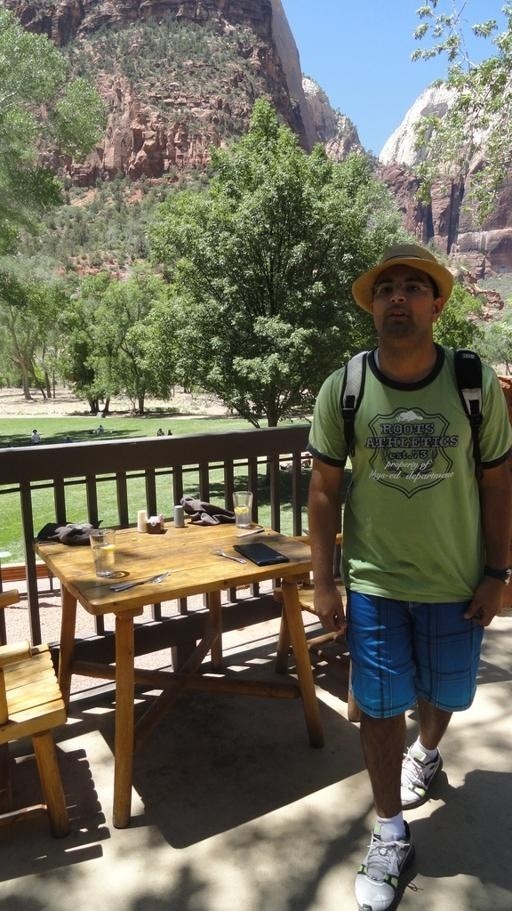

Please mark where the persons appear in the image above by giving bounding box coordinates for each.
[31,429,40,446]
[156,428,173,436]
[97,424,104,436]
[304,245,512,911]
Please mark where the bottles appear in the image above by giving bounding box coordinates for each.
[137,510,147,532]
[174,505,185,528]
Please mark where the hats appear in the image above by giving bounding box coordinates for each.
[351,244,455,313]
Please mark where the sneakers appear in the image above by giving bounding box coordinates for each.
[354,818,414,910]
[400,739,441,810]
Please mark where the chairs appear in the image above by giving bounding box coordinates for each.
[273,532,361,722]
[1,590,69,838]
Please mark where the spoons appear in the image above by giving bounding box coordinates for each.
[114,572,169,592]
[211,549,247,565]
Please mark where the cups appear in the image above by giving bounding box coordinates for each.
[232,491,253,529]
[89,529,115,578]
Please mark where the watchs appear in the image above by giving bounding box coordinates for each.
[484,568,512,585]
